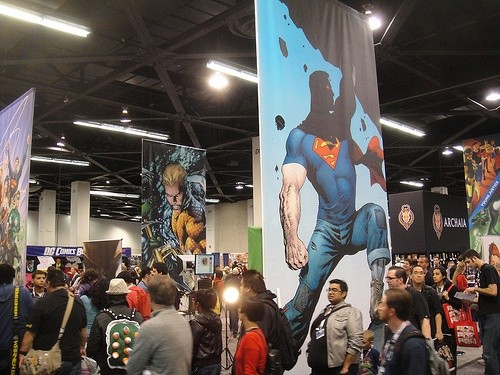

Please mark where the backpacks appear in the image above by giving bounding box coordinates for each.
[262,299,299,371]
[105,314,143,371]
[400,330,450,375]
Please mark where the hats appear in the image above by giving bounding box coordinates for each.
[105,280,131,295]
[233,267,241,275]
[223,266,230,270]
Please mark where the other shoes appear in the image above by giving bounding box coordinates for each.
[229,326,240,338]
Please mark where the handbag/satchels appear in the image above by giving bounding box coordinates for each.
[453,303,482,348]
[443,284,467,328]
[432,334,455,365]
[21,342,61,374]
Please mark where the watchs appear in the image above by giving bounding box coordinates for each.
[18,350,27,356]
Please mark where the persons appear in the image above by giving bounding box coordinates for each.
[380,248,500,375]
[0,258,279,375]
[358,330,381,375]
[306,279,363,375]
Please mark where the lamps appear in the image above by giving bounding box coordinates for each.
[380,114,427,138]
[0,0,94,38]
[74,120,171,143]
[204,57,257,83]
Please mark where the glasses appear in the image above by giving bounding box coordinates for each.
[385,276,397,280]
[326,288,343,293]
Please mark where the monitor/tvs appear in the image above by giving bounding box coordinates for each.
[195,254,215,275]
